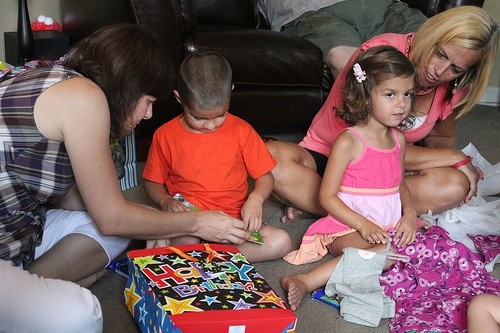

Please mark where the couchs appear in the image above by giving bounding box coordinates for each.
[131,0,484,133]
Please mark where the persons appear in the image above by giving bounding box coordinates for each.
[280,45,416,312]
[257,0,430,83]
[264,5,497,230]
[143,47,292,264]
[466,293,500,333]
[0,23,249,333]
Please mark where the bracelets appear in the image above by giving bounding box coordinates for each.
[451,156,473,169]
